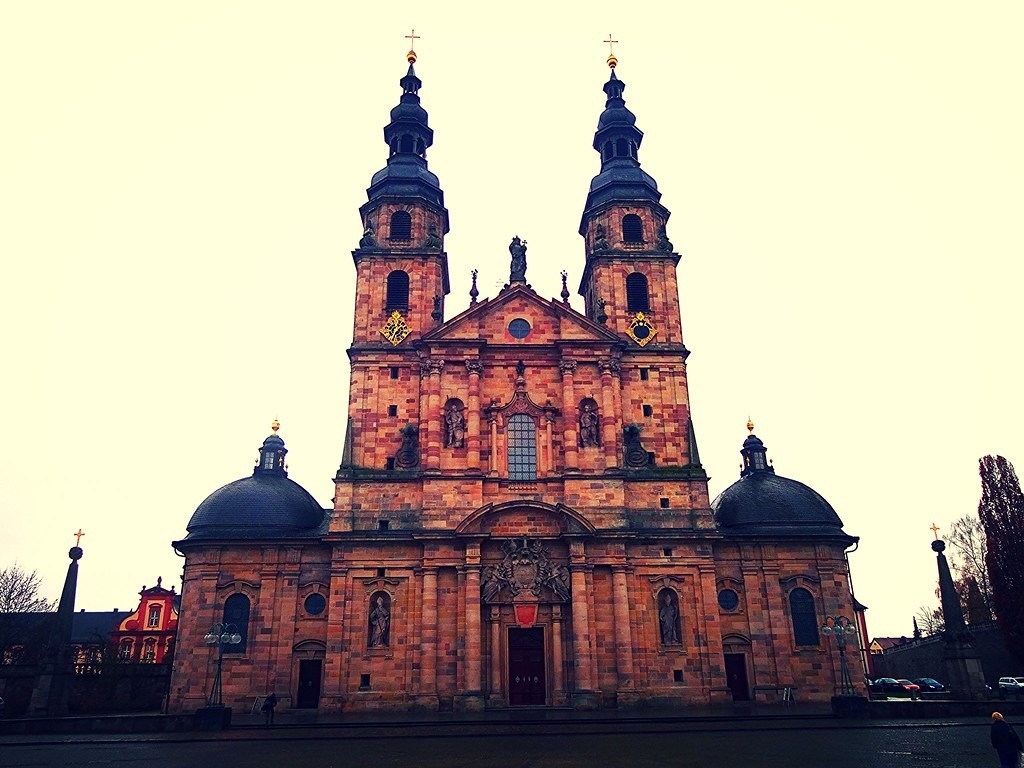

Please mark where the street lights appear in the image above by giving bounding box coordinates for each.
[821,615,871,718]
[194,625,242,732]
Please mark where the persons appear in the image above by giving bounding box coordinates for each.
[580,405,599,447]
[660,594,679,644]
[264,693,277,725]
[370,596,390,647]
[990,712,1024,768]
[445,405,464,448]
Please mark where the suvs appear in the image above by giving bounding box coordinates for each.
[998,677,1024,694]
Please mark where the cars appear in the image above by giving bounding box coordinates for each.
[895,679,921,693]
[913,678,946,692]
[874,678,903,692]
